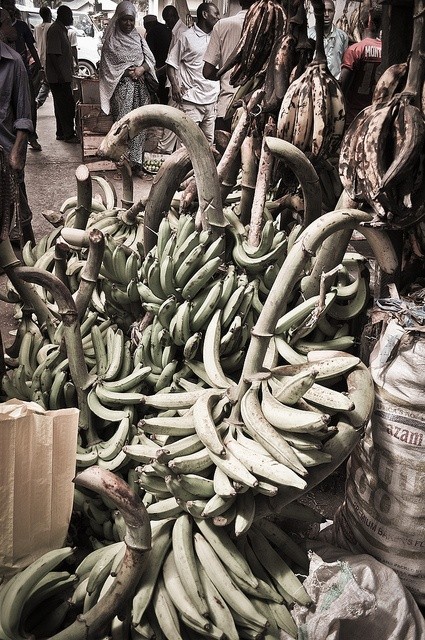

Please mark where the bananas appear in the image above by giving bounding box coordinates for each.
[336,61,425,232]
[276,59,348,159]
[219,0,288,82]
[0,176,373,640]
[261,27,300,106]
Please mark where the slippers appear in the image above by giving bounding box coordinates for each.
[63,137,80,143]
[55,135,63,140]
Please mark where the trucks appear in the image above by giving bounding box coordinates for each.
[24,7,105,80]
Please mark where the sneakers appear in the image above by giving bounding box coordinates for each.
[29,139,41,150]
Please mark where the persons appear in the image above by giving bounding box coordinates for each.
[34,7,53,105]
[308,0,349,84]
[203,0,254,152]
[163,5,187,52]
[0,41,37,248]
[99,0,153,180]
[65,26,77,63]
[46,5,82,142]
[4,5,41,151]
[143,15,171,105]
[339,5,382,120]
[167,3,220,150]
